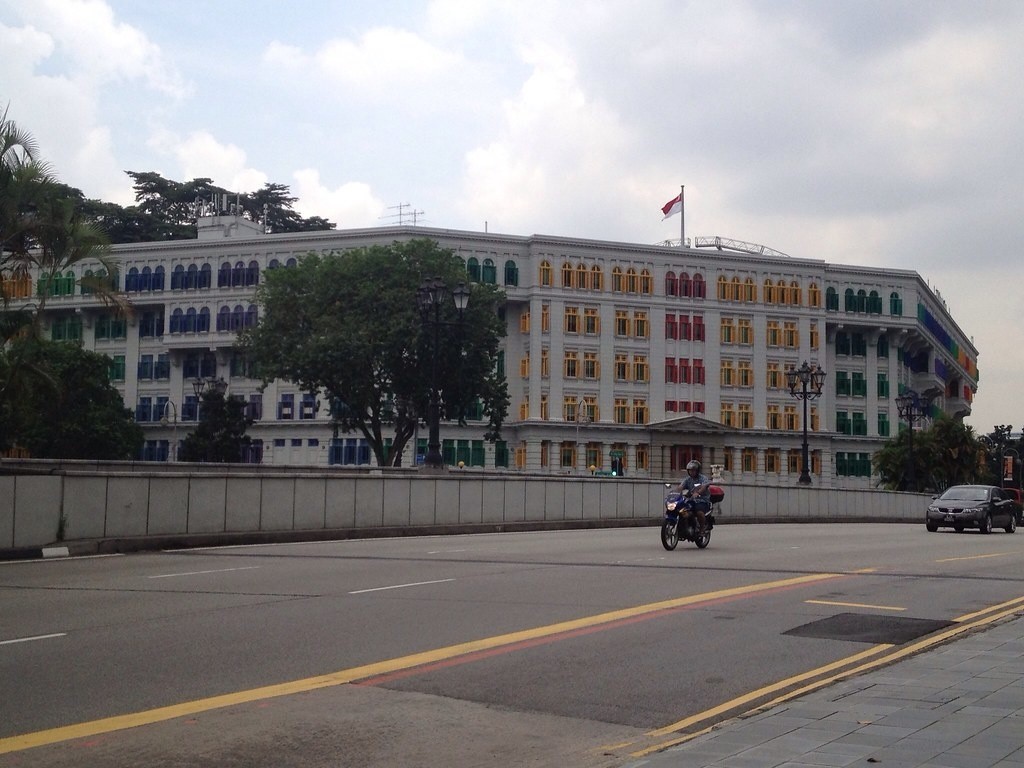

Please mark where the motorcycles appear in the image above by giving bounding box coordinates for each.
[659,483,725,551]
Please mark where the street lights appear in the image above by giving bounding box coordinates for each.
[1000,447,1022,488]
[895,389,929,493]
[159,400,177,461]
[575,397,591,476]
[786,360,828,487]
[191,374,230,462]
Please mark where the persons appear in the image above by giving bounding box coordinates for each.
[668,459,712,536]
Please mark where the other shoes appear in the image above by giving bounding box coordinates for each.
[699,526,705,536]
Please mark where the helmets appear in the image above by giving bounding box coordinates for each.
[686,460,701,477]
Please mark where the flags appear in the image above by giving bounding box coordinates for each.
[662,191,683,221]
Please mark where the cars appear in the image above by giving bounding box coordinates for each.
[1002,488,1024,527]
[925,484,1017,534]
